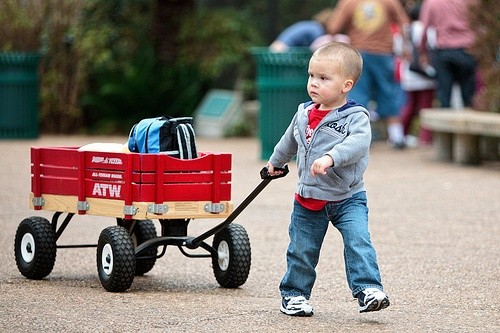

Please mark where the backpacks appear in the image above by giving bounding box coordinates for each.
[127,116,199,183]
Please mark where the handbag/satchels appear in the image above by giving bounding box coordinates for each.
[409,42,438,78]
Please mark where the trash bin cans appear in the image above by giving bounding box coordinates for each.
[250,45,313,162]
[0,51,43,138]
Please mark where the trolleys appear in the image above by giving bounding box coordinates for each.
[14,145,290,293]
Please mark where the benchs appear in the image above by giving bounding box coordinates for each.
[417,108,500,165]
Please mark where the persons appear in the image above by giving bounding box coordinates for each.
[268,0,487,150]
[266,41,390,317]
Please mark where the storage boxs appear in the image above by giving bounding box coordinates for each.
[24,146,233,221]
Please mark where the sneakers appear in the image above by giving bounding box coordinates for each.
[358,288,389,312]
[280,295,314,317]
